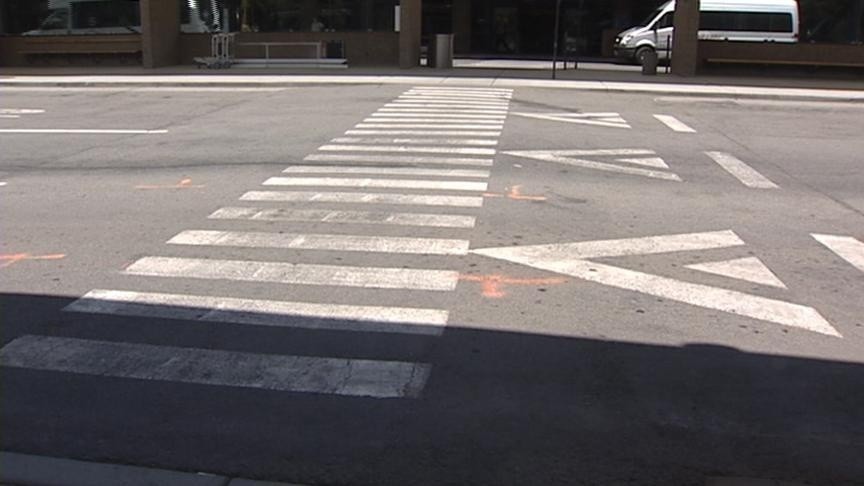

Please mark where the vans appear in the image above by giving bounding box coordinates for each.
[612,0,800,65]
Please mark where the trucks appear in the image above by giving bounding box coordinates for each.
[23,0,221,36]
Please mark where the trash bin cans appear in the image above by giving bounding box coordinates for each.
[427,33,454,67]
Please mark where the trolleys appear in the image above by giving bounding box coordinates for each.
[193,33,230,69]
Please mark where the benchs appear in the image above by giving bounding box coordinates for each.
[705,59,864,77]
[12,44,141,65]
